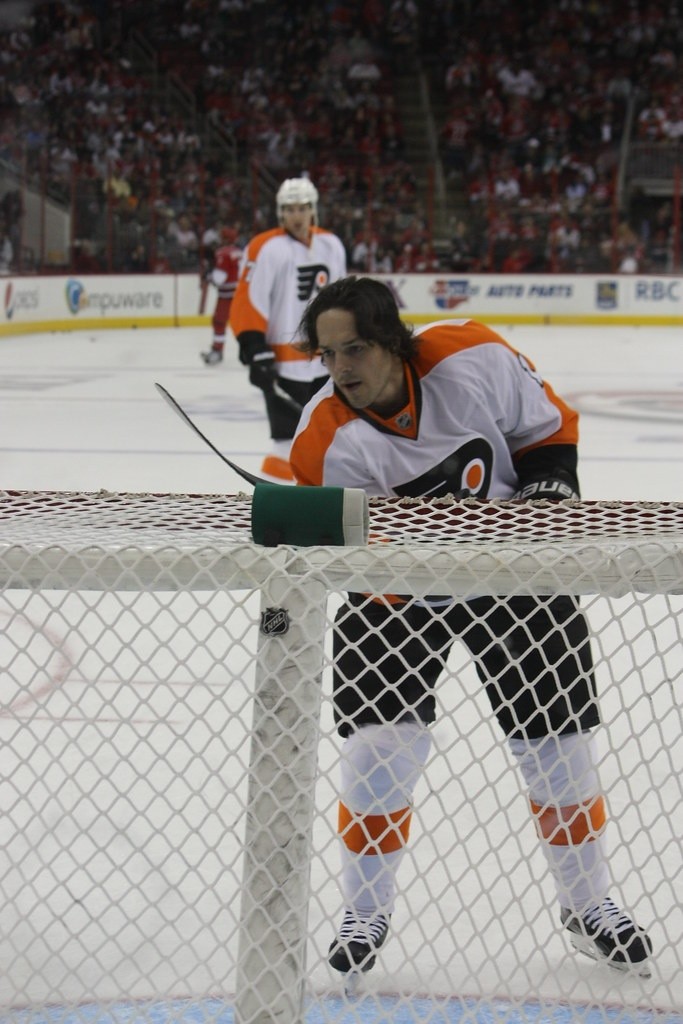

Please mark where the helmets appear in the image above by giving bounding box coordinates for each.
[275,177,319,206]
[220,226,238,243]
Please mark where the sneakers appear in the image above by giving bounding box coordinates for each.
[202,347,223,364]
[559,897,654,977]
[326,908,390,996]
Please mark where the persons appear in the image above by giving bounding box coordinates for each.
[0,0,683,274]
[288,276,652,996]
[202,227,244,365]
[232,174,347,485]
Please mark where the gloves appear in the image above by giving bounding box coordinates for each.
[246,347,282,392]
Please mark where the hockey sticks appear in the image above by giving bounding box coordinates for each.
[155,380,276,489]
[271,380,304,413]
[195,257,211,315]
